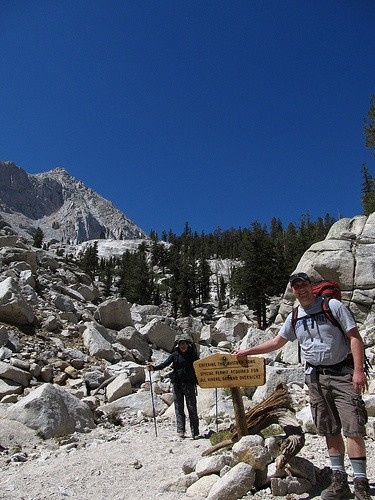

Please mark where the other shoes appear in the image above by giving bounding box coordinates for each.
[192,432,197,437]
[179,431,184,437]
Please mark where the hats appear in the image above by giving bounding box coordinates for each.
[290,272,311,288]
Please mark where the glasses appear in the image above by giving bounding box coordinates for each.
[289,273,308,281]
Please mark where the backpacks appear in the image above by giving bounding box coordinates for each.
[171,333,198,368]
[292,280,345,338]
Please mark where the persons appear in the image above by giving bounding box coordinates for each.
[230,272,372,500]
[145,333,202,439]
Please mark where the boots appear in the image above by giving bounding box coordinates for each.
[353,477,373,500]
[321,471,351,500]
